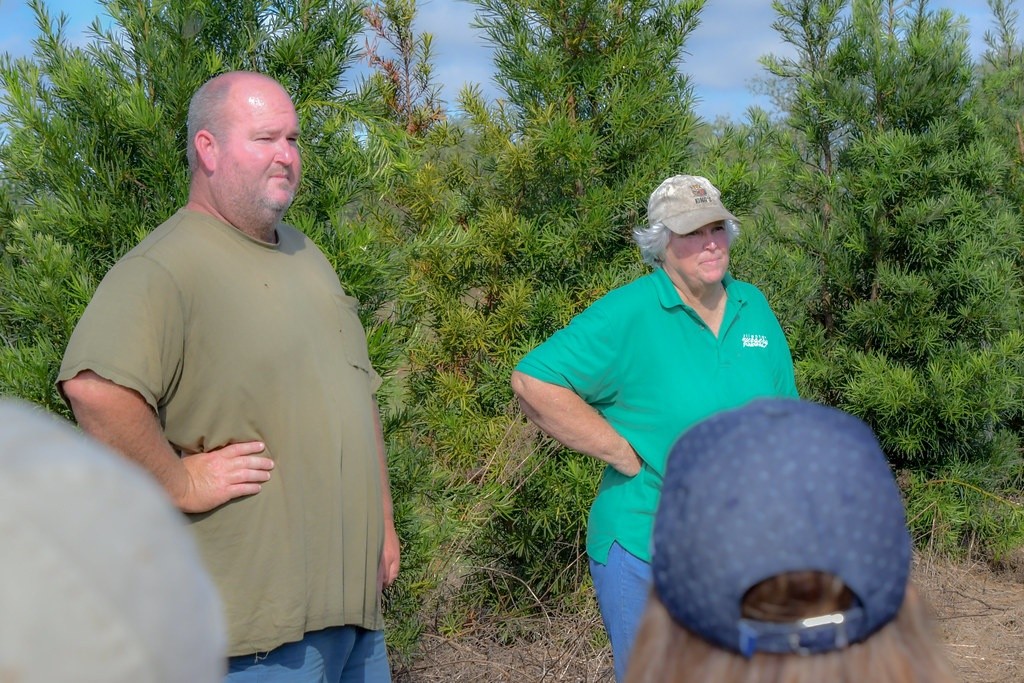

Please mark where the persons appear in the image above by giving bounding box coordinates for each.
[0,397,228,683]
[622,398,958,683]
[513,175,799,683]
[56,74,400,683]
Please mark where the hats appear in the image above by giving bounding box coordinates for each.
[653,398,910,659]
[648,174,743,235]
